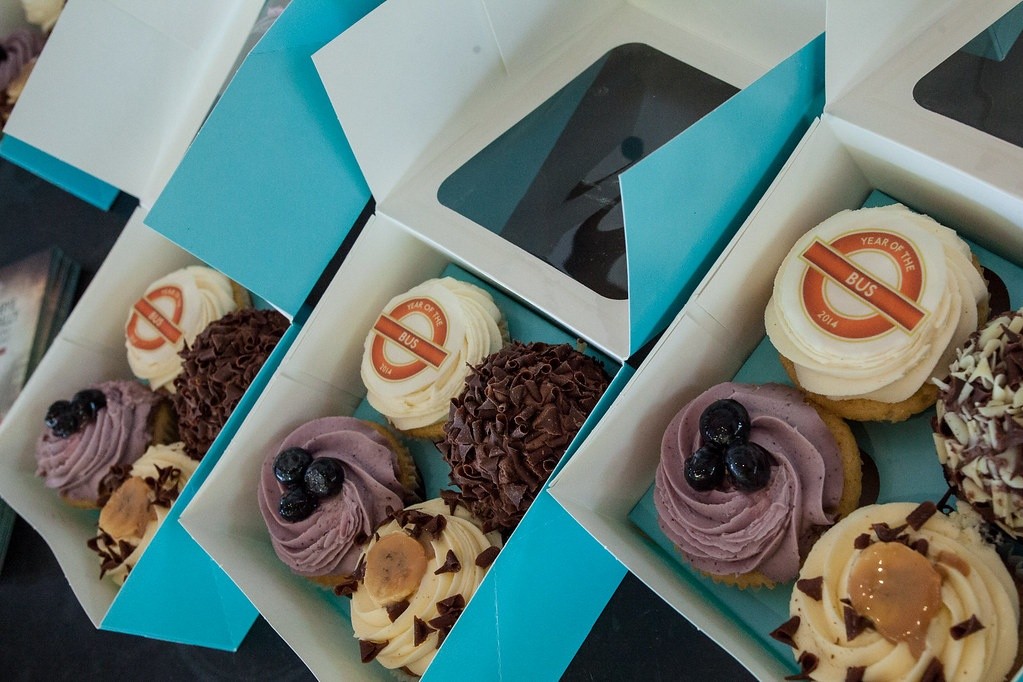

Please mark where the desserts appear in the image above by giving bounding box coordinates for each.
[34,265,617,678]
[656,202,1023,682]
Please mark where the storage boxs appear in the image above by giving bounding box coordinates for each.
[0,0,1023,682]
